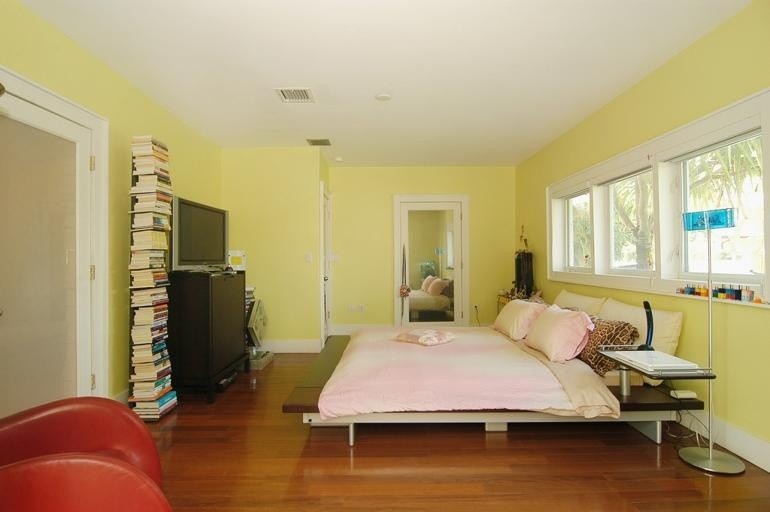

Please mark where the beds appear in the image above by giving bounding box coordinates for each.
[409,278,454,320]
[282,336,704,446]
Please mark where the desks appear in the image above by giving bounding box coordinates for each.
[165,270,247,404]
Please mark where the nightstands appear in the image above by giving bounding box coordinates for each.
[497,295,508,317]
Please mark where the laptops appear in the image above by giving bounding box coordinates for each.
[615,350,698,372]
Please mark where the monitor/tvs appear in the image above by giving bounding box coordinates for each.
[169,195,229,273]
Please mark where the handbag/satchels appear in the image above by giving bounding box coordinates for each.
[400,284,411,298]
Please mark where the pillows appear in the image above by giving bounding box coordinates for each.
[524,305,594,362]
[576,315,639,376]
[491,299,544,341]
[529,291,578,310]
[421,275,448,296]
[599,298,682,356]
[552,290,605,315]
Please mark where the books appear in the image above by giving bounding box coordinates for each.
[127,133,182,425]
[243,284,257,353]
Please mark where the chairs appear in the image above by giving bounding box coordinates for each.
[0,453,172,512]
[0,397,162,489]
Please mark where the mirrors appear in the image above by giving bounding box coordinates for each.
[394,195,469,327]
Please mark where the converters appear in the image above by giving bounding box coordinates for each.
[670,389,697,399]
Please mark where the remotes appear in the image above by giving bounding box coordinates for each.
[214,271,237,275]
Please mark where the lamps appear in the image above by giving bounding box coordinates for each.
[677,208,746,475]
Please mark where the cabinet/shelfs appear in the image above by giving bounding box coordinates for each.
[128,136,180,421]
